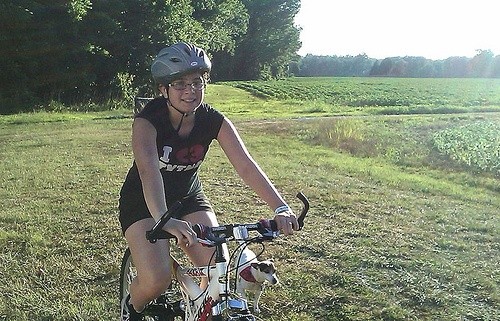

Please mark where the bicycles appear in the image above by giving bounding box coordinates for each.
[119,192,310,321]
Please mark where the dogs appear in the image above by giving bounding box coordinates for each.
[232,239,278,314]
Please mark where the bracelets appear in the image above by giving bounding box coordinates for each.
[274,205,291,213]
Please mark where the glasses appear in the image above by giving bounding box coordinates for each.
[164,80,204,90]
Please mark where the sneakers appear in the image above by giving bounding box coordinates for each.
[120,294,146,321]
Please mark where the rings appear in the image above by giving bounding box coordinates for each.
[288,222,292,225]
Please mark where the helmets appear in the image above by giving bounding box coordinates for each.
[150,43,210,83]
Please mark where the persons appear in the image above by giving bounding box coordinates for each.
[118,41,300,321]
